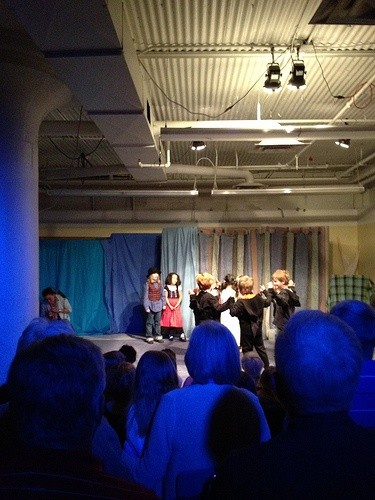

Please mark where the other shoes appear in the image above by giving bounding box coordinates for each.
[155,336,164,343]
[169,335,174,341]
[145,337,155,344]
[179,335,186,342]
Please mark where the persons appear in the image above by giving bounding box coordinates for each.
[0,269,375,500]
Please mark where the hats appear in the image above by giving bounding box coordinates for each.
[146,266,162,278]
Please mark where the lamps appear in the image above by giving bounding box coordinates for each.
[262,48,283,93]
[335,139,351,148]
[287,48,307,89]
[191,141,206,150]
